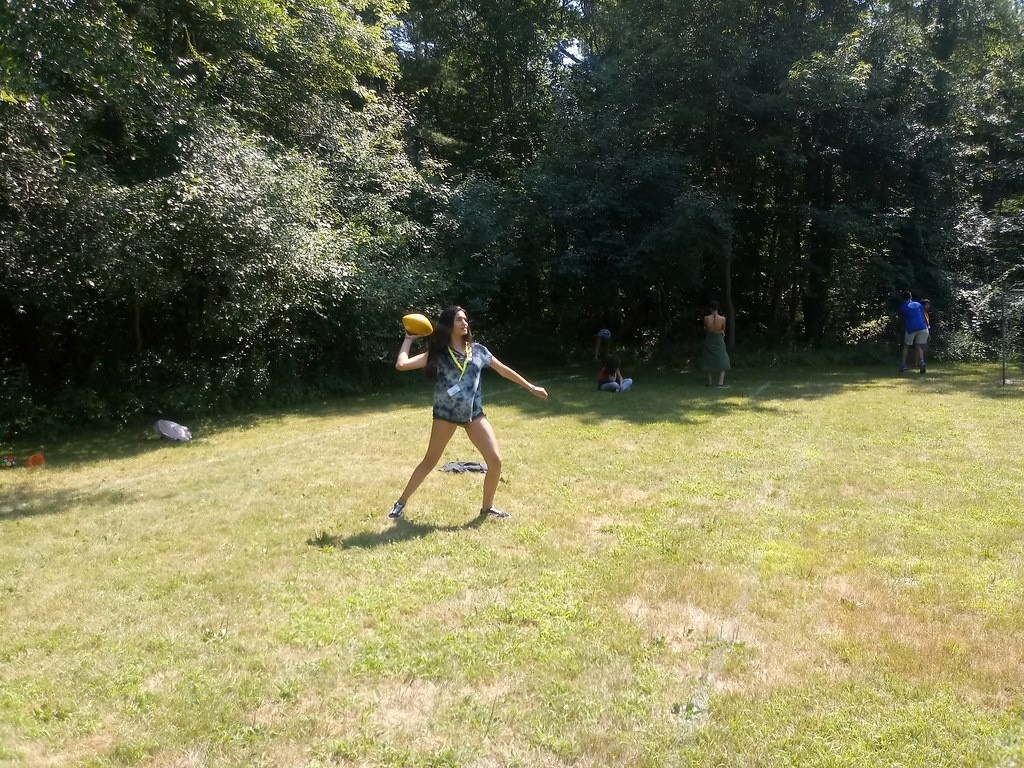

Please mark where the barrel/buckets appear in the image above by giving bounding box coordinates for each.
[24,454,45,467]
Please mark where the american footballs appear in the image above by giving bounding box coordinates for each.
[402,314,433,337]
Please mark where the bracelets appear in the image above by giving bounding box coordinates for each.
[529,385,535,392]
[405,336,413,340]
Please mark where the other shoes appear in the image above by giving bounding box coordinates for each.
[919,363,926,374]
[717,384,728,389]
[897,366,907,373]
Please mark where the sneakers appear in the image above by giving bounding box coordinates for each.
[388,503,404,519]
[481,504,511,519]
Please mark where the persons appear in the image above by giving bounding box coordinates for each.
[387,306,548,520]
[703,302,731,389]
[599,358,633,391]
[900,291,931,374]
